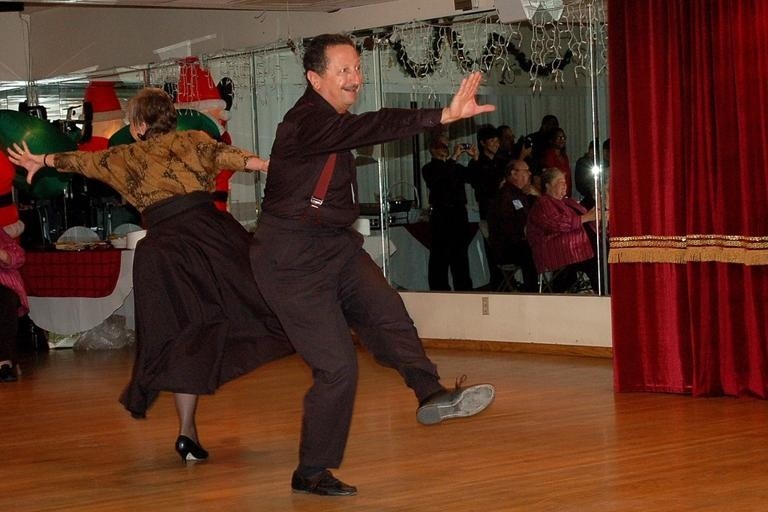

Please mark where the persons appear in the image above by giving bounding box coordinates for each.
[527,168,605,296]
[0,229,28,381]
[6,88,270,464]
[498,160,541,293]
[422,134,479,291]
[469,115,610,220]
[250,34,497,496]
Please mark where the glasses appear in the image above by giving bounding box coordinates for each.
[555,136,565,140]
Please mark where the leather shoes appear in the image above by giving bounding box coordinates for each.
[292,467,358,496]
[416,384,495,426]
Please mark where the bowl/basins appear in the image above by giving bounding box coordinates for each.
[111,236,126,249]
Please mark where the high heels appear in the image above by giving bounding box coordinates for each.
[174,434,208,464]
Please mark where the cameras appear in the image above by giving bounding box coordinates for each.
[518,136,534,148]
[462,144,472,152]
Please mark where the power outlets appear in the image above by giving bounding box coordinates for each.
[481,297,490,315]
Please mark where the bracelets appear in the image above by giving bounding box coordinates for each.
[44,154,48,167]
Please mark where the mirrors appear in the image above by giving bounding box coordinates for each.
[31,62,143,135]
[0,87,26,111]
[143,51,257,232]
[373,3,608,298]
[251,28,385,278]
